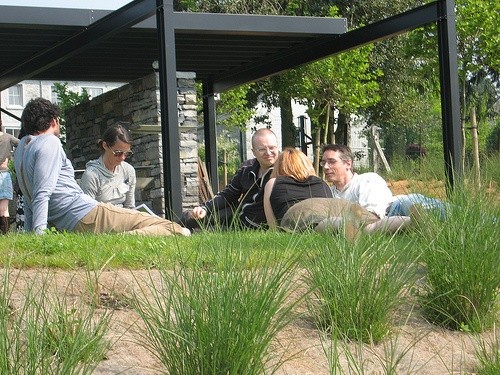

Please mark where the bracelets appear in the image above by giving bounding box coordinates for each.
[202,206,207,211]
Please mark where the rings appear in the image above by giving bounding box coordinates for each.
[6,166,7,167]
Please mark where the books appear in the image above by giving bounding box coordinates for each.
[134,203,161,217]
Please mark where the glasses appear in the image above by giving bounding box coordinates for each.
[320,160,346,166]
[107,143,133,158]
[253,146,278,154]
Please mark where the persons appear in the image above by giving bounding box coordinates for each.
[13,97,191,237]
[0,121,21,236]
[320,143,500,225]
[175,128,281,234]
[263,147,437,246]
[80,123,136,208]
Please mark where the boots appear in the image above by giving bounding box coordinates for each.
[1,216,9,234]
